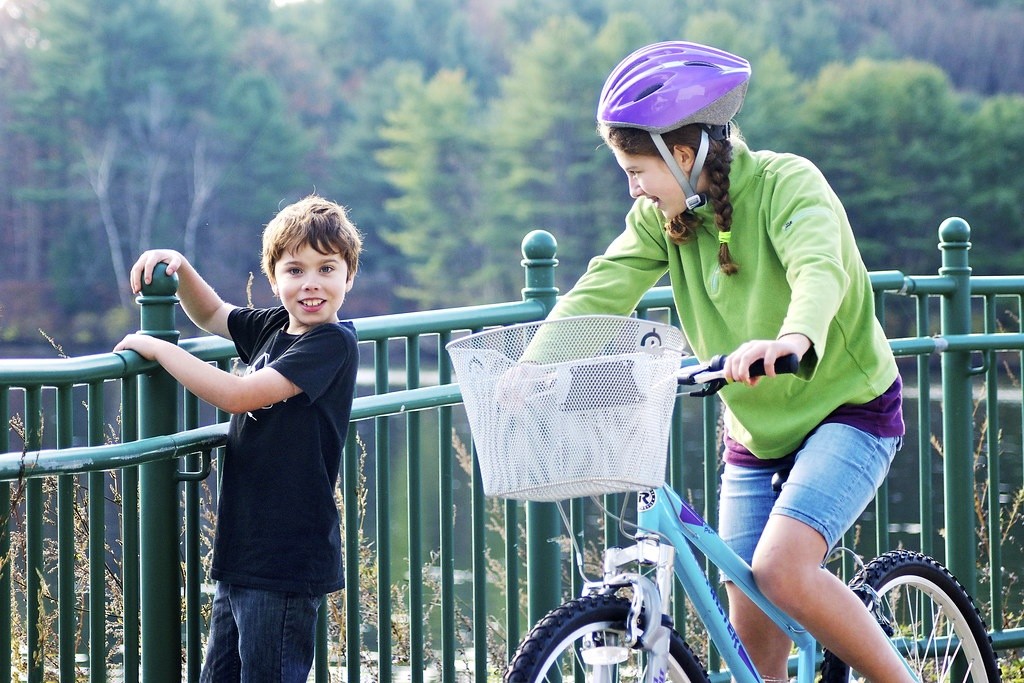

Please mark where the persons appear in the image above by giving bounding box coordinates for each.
[114,196,360,683]
[496,41,915,683]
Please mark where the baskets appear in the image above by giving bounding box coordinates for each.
[444,315,689,505]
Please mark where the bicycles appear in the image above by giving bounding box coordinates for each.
[444,311,1007,683]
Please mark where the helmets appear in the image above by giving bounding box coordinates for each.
[596,39,753,213]
